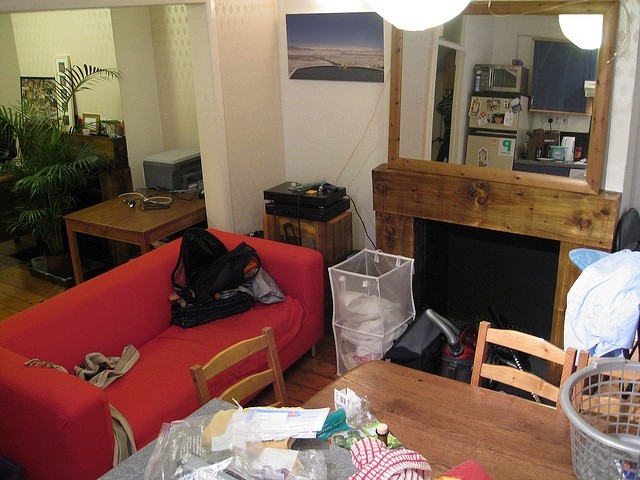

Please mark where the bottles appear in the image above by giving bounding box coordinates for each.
[535,147,542,158]
[529,95,535,109]
[375,423,390,447]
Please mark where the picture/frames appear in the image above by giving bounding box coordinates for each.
[82,114,99,136]
[19,76,58,127]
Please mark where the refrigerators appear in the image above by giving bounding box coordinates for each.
[465,95,529,171]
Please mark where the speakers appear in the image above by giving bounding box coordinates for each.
[54,55,75,134]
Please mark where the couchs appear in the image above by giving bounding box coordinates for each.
[0,227,326,480]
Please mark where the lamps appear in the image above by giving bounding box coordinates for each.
[357,1,474,32]
[557,12,604,52]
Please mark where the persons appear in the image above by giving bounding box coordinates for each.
[492,114,504,123]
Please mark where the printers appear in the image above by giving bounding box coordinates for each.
[142,148,202,193]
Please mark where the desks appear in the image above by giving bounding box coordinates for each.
[299,358,640,480]
[62,186,205,286]
[263,210,354,267]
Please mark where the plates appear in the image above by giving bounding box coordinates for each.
[536,157,555,162]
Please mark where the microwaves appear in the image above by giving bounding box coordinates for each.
[473,63,530,95]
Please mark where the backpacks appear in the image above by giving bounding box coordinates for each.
[171,227,229,302]
[195,241,260,304]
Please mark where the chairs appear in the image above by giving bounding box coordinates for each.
[191,327,291,415]
[576,349,640,423]
[469,319,579,408]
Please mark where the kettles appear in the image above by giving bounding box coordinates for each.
[560,136,576,161]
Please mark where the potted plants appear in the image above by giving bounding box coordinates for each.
[0,61,126,276]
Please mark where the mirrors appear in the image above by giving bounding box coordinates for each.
[380,2,620,195]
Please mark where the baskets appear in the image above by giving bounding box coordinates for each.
[558,356,640,480]
